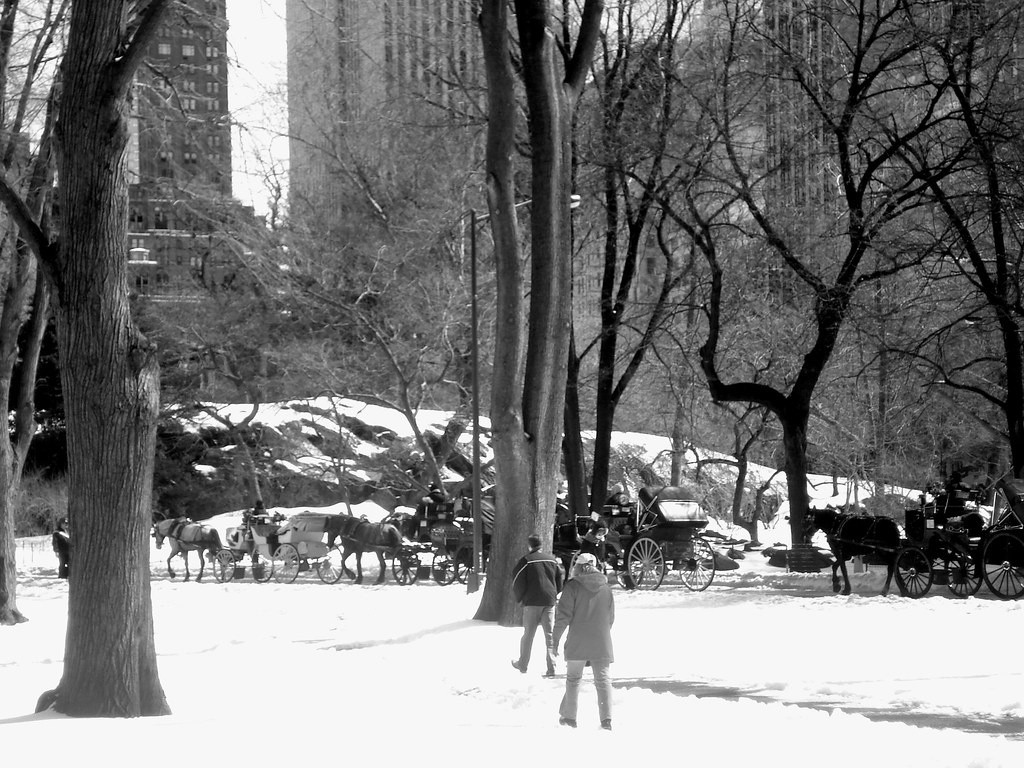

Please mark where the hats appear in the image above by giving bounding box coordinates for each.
[576,553,596,567]
[429,484,438,491]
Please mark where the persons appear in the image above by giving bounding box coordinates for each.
[603,482,631,505]
[53,518,70,579]
[512,533,564,678]
[233,500,269,543]
[552,519,617,730]
[408,482,449,539]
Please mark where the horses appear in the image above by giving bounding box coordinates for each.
[151,518,229,584]
[323,513,408,586]
[803,504,904,598]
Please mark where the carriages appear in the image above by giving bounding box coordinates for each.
[440,467,717,592]
[299,509,463,586]
[799,478,1024,599]
[150,511,346,584]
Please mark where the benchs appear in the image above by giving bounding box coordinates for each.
[278,513,329,542]
[251,524,282,545]
[418,498,456,522]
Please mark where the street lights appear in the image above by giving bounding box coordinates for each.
[468,192,581,574]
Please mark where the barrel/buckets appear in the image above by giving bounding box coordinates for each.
[951,567,968,584]
[433,570,446,580]
[252,565,265,579]
[418,567,430,580]
[622,574,637,590]
[232,568,245,579]
[932,569,949,585]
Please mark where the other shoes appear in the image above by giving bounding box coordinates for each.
[601,719,611,730]
[512,660,526,673]
[559,716,577,728]
[542,674,555,679]
